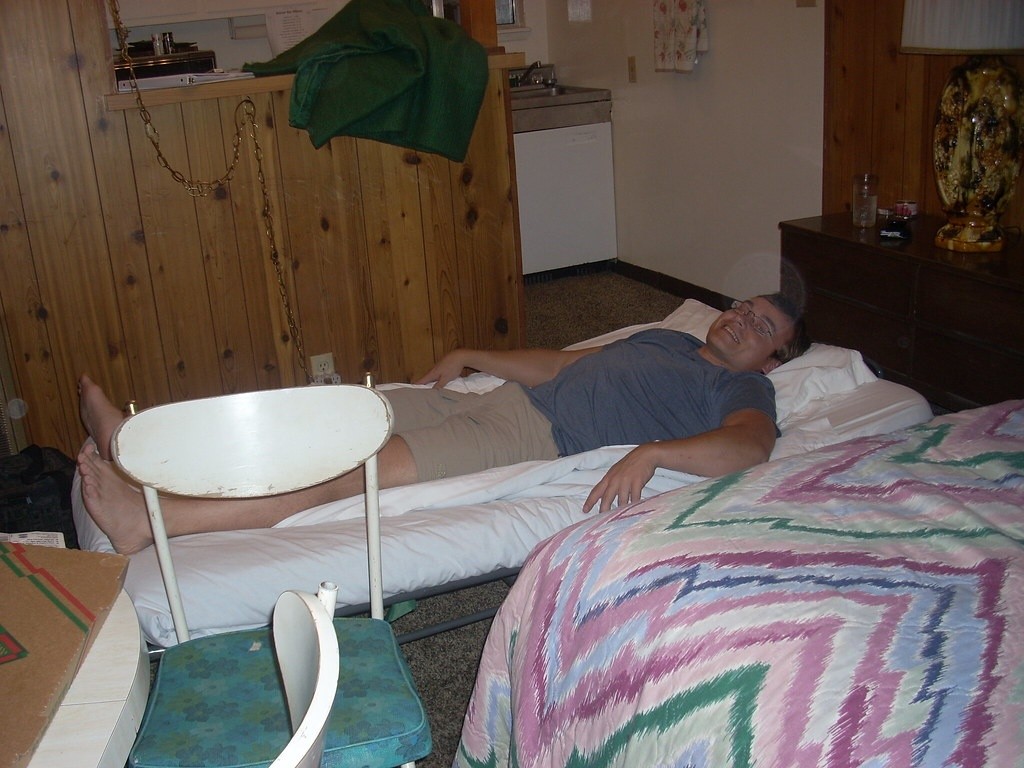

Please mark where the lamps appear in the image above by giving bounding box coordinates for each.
[897,0,1024,254]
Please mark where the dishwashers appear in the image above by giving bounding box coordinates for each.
[513,122,617,282]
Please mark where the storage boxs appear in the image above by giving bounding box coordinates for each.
[0,540,130,768]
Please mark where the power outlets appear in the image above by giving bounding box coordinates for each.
[628,55,637,84]
[309,352,341,385]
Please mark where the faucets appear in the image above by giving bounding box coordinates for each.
[520,60,541,84]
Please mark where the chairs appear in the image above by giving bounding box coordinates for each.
[268,581,339,768]
[113,372,434,768]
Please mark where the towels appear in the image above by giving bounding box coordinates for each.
[240,0,489,162]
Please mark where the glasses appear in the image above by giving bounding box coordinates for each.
[731,299,773,337]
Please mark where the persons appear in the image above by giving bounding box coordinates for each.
[77,290,811,555]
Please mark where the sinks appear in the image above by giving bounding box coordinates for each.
[511,84,611,111]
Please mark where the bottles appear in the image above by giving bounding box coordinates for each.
[151,31,175,56]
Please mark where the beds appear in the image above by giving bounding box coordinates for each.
[451,402,1024,768]
[70,321,934,661]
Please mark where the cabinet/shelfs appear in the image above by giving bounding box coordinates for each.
[777,211,1024,412]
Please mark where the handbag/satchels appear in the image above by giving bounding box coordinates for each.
[0,443,82,550]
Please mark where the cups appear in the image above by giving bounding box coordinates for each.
[852,170,878,228]
[895,199,917,216]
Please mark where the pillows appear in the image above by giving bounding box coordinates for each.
[656,299,879,426]
